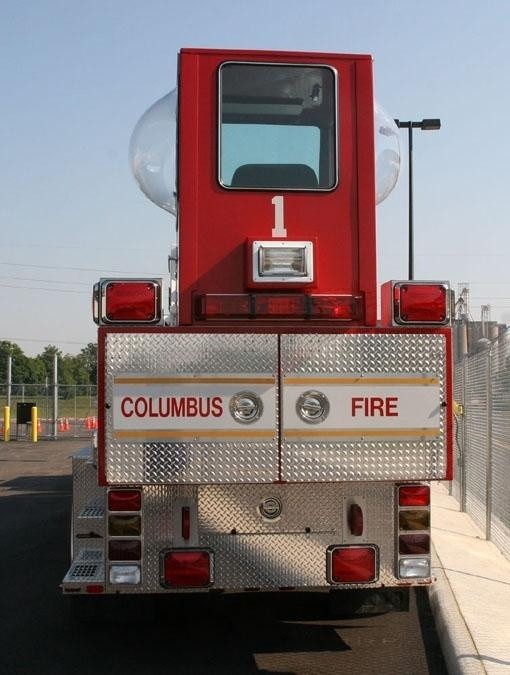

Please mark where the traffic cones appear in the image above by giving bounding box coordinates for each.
[37,416,97,433]
[0,420,5,433]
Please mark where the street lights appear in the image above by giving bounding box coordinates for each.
[380,118,441,279]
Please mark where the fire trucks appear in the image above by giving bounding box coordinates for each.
[58,46,456,614]
